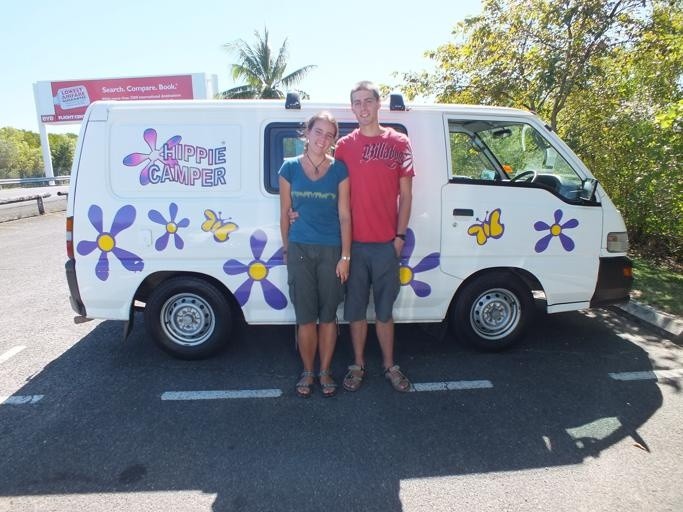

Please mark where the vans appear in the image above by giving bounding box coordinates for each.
[66,93,634,362]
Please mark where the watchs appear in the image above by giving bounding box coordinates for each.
[395,235,407,241]
[341,256,350,261]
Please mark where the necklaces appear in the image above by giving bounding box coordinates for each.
[307,155,326,174]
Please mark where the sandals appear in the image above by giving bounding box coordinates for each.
[342,364,368,392]
[383,363,411,393]
[317,369,336,396]
[294,371,316,398]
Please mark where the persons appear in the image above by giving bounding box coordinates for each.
[278,112,352,398]
[288,81,414,396]
